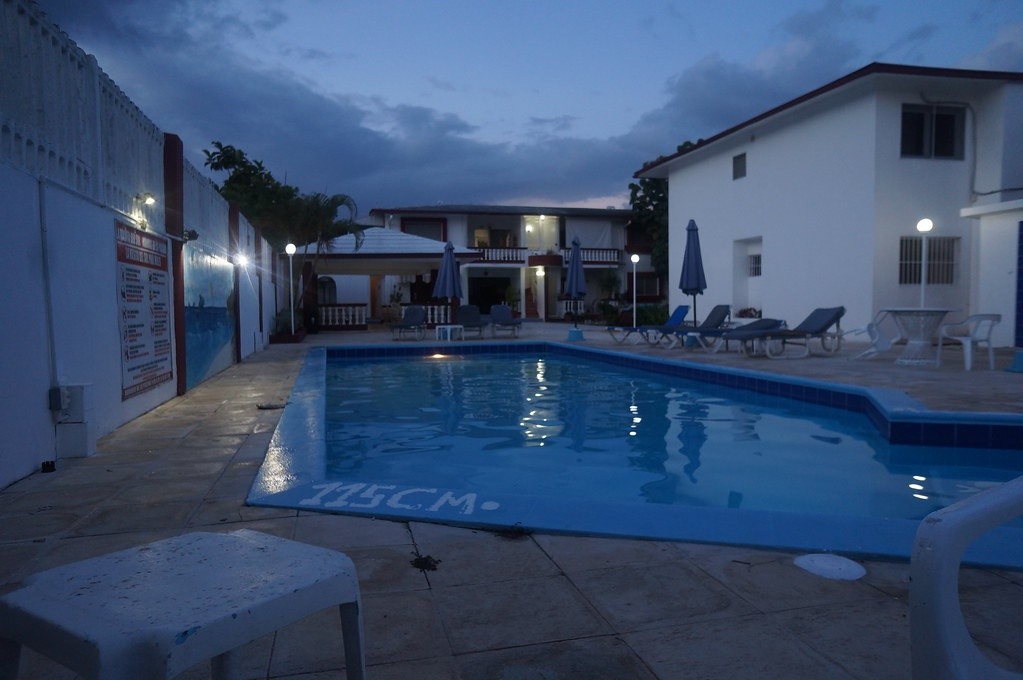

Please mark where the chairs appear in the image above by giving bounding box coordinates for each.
[676,318,788,355]
[639,304,732,349]
[841,308,903,362]
[489,305,522,339]
[722,306,847,359]
[604,305,691,345]
[455,305,488,339]
[936,313,1002,371]
[388,305,428,341]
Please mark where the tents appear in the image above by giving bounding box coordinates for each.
[278,225,486,278]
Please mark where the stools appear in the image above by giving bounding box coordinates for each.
[1,529,366,680]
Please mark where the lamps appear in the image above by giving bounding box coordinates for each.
[137,192,156,206]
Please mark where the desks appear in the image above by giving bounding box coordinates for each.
[435,324,465,342]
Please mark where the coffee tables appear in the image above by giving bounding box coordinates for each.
[879,306,962,365]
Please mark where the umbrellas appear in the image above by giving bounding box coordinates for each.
[563,234,587,329]
[431,240,464,325]
[678,218,708,328]
